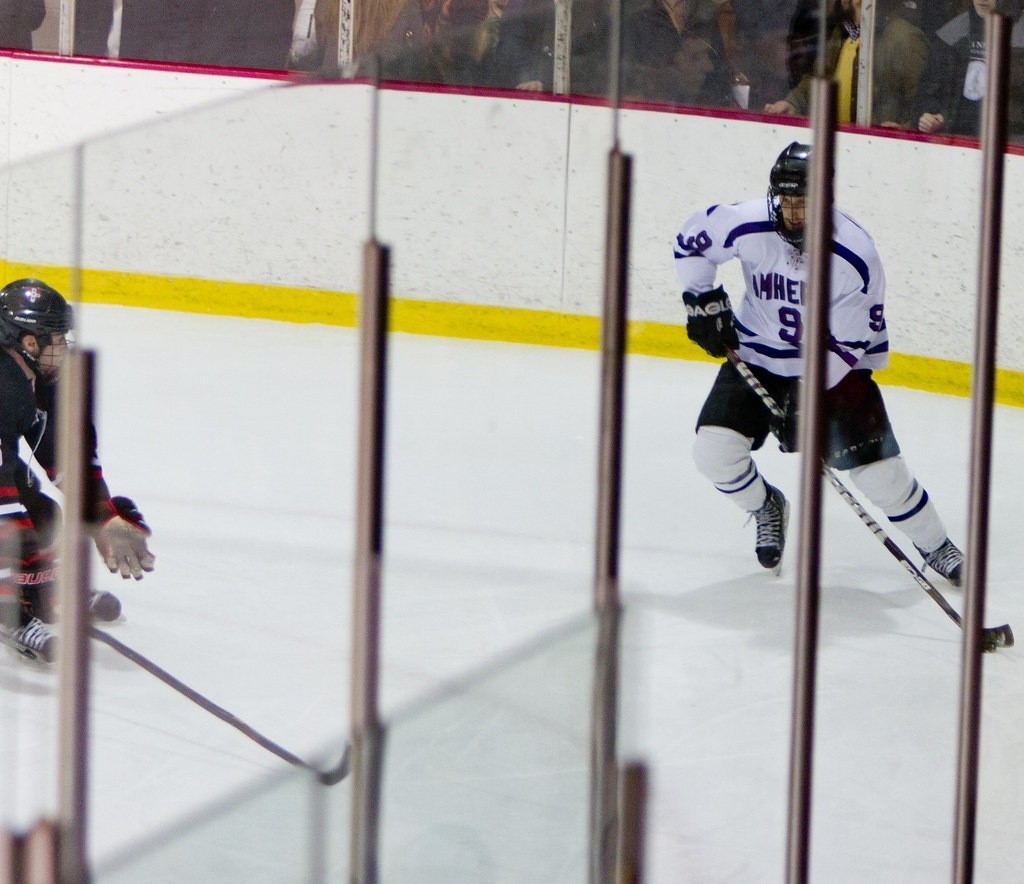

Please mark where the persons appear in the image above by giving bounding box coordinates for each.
[0,0,1024,146]
[0,278,155,667]
[671,141,965,591]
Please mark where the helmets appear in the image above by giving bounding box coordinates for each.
[0,278,76,385]
[765,142,812,247]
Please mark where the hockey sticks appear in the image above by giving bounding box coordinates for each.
[88,625,353,788]
[724,345,1015,649]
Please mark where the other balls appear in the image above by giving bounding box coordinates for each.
[982,640,996,653]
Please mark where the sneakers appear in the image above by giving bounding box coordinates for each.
[744,475,792,577]
[1,614,64,666]
[86,587,130,626]
[915,540,969,591]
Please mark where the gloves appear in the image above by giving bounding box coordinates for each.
[777,382,804,453]
[680,284,758,357]
[94,516,154,580]
